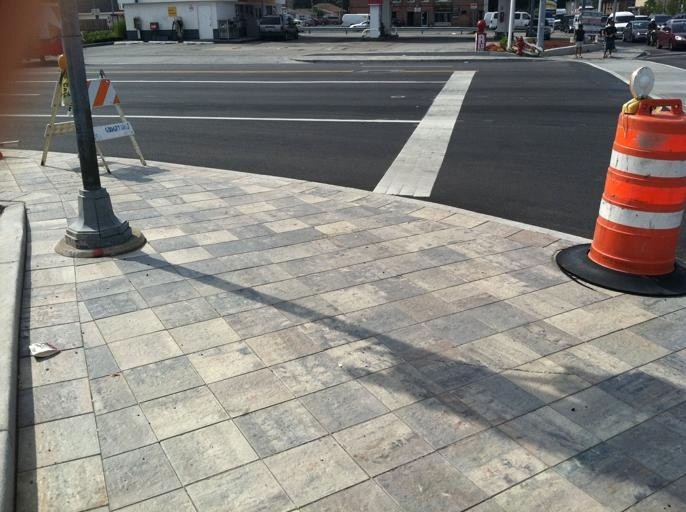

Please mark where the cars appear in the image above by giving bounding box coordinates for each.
[350,20,370,30]
[483,6,686,53]
[293,15,342,29]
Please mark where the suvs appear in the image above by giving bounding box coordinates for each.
[258,13,298,41]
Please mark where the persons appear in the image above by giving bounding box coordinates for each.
[602,21,618,58]
[647,18,658,47]
[574,24,586,58]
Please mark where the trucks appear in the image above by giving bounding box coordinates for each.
[16,3,66,62]
[341,13,370,28]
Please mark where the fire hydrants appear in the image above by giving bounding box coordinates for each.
[515,34,525,57]
